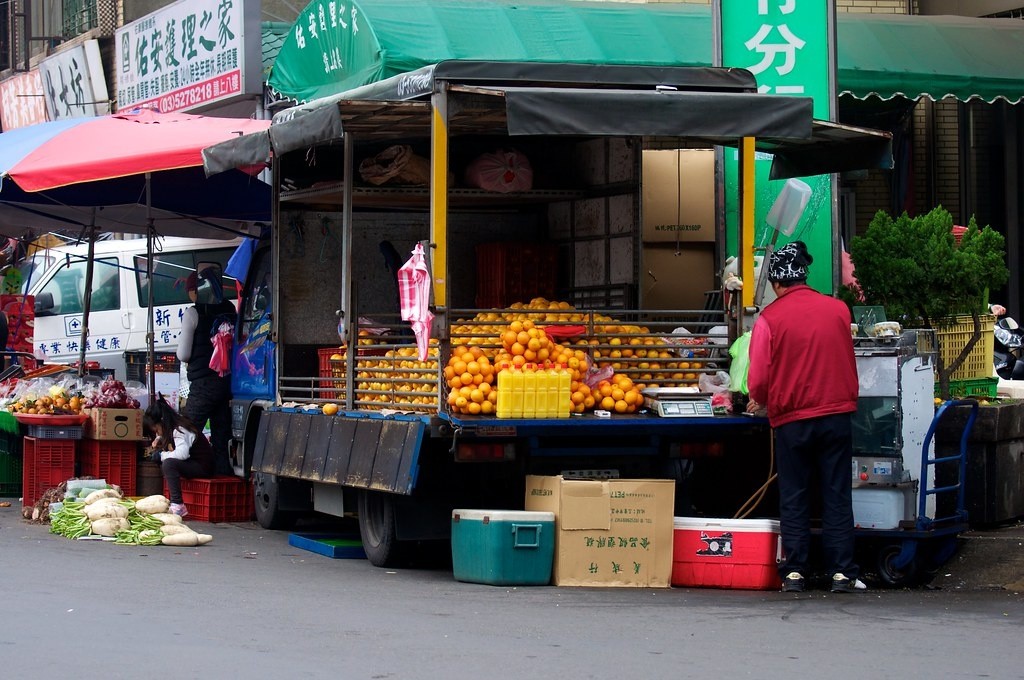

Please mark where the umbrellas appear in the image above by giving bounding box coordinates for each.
[0,106,274,408]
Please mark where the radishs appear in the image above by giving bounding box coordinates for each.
[84,489,214,546]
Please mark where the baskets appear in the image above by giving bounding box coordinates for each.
[28,425,82,440]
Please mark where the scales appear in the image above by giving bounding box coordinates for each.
[640,387,714,417]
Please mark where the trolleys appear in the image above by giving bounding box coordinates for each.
[809,399,988,589]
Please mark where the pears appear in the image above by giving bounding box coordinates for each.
[7,394,87,416]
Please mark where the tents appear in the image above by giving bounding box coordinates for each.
[202,66,896,345]
[265,0,1024,340]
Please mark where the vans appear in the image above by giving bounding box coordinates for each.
[0,229,245,390]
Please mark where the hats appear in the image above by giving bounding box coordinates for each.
[185,271,197,292]
[768,241,813,282]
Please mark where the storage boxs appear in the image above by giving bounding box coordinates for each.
[162,475,251,523]
[80,407,151,440]
[81,437,136,498]
[449,508,557,588]
[671,516,781,592]
[0,409,74,506]
[920,316,998,381]
[524,473,675,588]
[316,346,389,405]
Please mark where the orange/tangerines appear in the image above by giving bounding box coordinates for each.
[322,297,701,415]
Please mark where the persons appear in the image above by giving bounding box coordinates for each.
[175,270,239,473]
[988,303,1007,316]
[142,401,220,518]
[747,241,859,591]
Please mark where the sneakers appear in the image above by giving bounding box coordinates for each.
[831,573,868,593]
[169,504,188,517]
[782,572,804,592]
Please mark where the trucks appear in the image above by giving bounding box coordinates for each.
[194,58,897,570]
